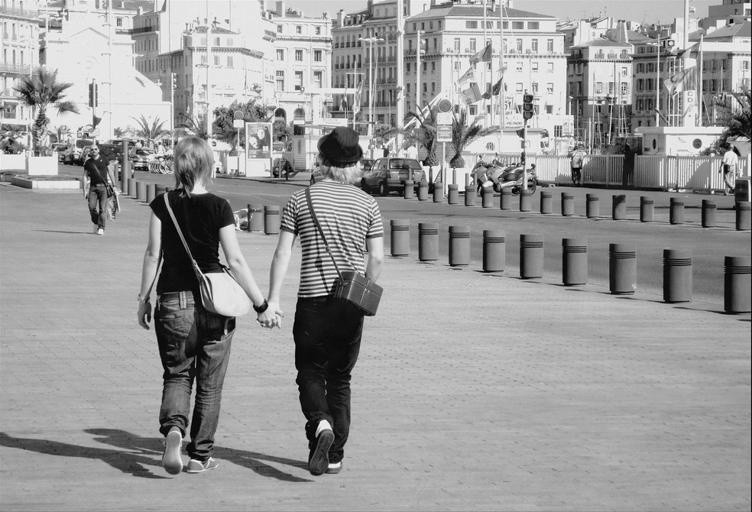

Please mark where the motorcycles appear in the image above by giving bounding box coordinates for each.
[469,154,523,191]
[476,158,538,196]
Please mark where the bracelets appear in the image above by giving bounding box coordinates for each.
[136,293,152,305]
[253,297,268,313]
[112,184,117,188]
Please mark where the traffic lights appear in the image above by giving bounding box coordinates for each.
[524,94,534,120]
[515,128,524,140]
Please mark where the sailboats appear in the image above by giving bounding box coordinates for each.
[580,0,751,188]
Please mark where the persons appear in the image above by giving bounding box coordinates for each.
[619,142,636,186]
[135,134,285,476]
[156,142,166,154]
[255,124,387,478]
[567,145,584,184]
[82,144,117,236]
[718,143,739,197]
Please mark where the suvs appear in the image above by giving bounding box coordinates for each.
[65,139,97,164]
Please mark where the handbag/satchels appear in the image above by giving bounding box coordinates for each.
[334,272,383,316]
[200,273,250,317]
[106,183,114,198]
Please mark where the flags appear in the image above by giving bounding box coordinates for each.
[468,45,493,64]
[462,83,483,106]
[481,77,504,101]
[453,66,475,89]
[662,65,698,98]
[675,42,700,59]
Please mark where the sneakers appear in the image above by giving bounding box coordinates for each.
[308,419,343,475]
[162,426,219,474]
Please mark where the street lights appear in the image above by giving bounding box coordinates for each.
[567,96,574,115]
[167,71,179,149]
[343,71,366,130]
[416,29,427,115]
[358,37,385,132]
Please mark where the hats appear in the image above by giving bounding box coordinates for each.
[317,127,362,163]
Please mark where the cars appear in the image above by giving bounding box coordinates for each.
[53,145,69,162]
[363,157,427,199]
[129,147,157,171]
[362,159,377,174]
